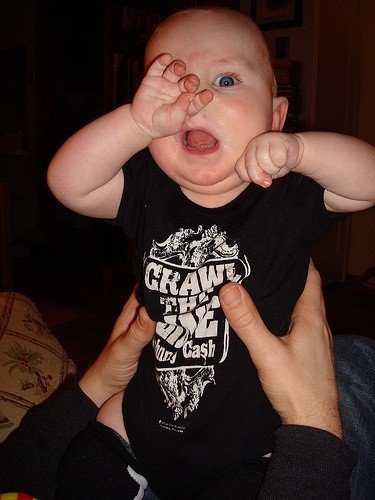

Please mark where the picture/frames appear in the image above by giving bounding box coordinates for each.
[251,0,303,32]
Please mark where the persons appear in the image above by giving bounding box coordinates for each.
[0,258,375,500]
[48,5,375,500]
